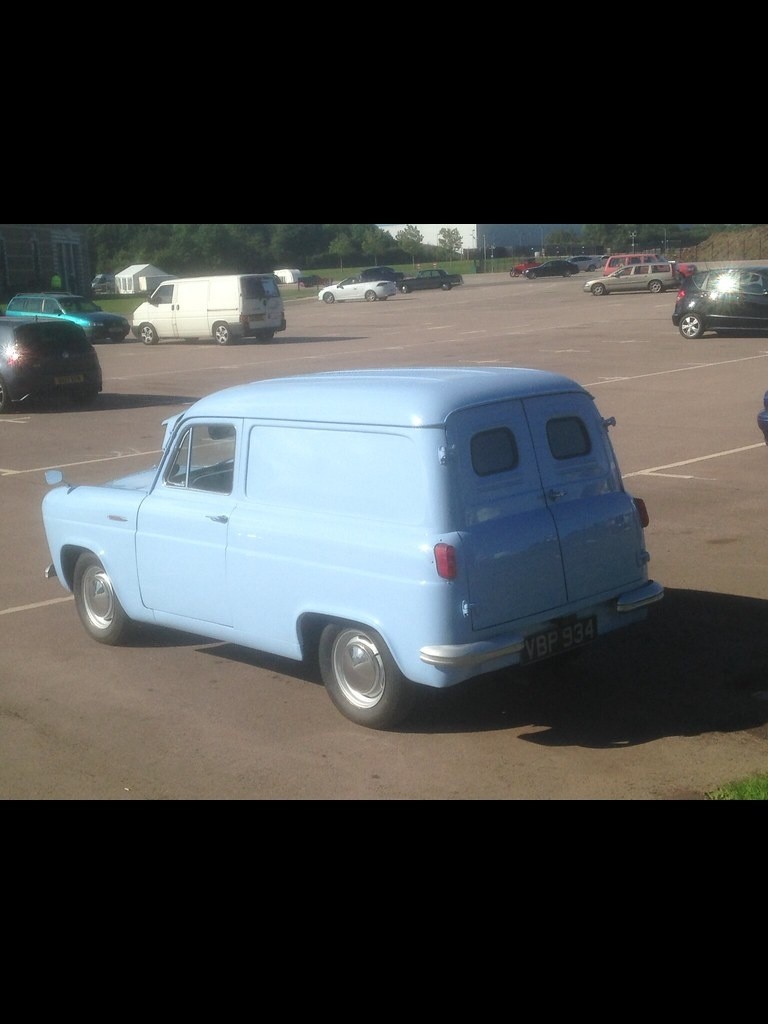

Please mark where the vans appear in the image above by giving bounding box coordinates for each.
[129,273,287,344]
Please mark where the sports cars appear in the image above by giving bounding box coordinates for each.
[317,277,396,302]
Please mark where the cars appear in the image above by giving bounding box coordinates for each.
[582,263,684,295]
[511,258,542,273]
[672,264,768,340]
[395,268,464,293]
[0,315,105,412]
[353,266,406,283]
[521,259,579,279]
[565,255,601,272]
[38,367,666,734]
[92,273,111,291]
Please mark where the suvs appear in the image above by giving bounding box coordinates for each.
[6,291,132,345]
[602,252,697,279]
